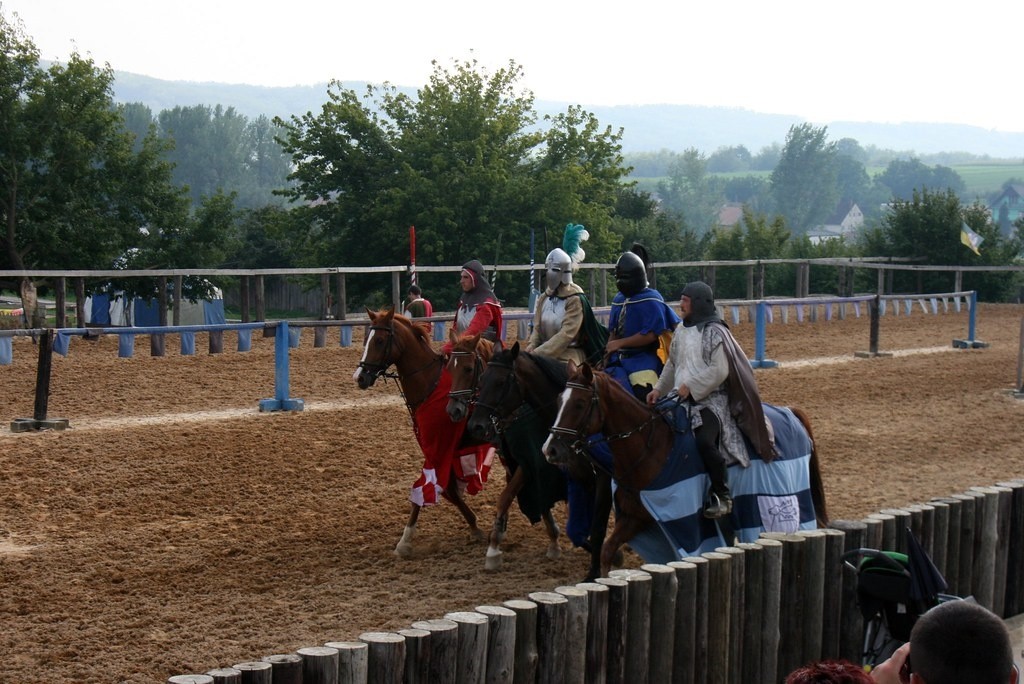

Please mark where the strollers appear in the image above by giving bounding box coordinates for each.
[834,525,972,675]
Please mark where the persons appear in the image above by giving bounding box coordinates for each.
[433,259,502,436]
[646,280,732,518]
[594,250,666,404]
[869,600,1018,684]
[404,285,433,333]
[525,247,591,380]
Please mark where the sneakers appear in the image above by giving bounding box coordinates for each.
[705,495,733,517]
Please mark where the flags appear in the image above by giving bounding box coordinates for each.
[960,221,985,256]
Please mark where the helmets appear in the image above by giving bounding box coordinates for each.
[614,252,649,295]
[545,247,574,291]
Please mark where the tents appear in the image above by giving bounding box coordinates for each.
[83,247,226,327]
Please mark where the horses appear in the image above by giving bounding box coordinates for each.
[445,327,569,575]
[352,305,504,557]
[541,359,829,583]
[465,339,624,584]
